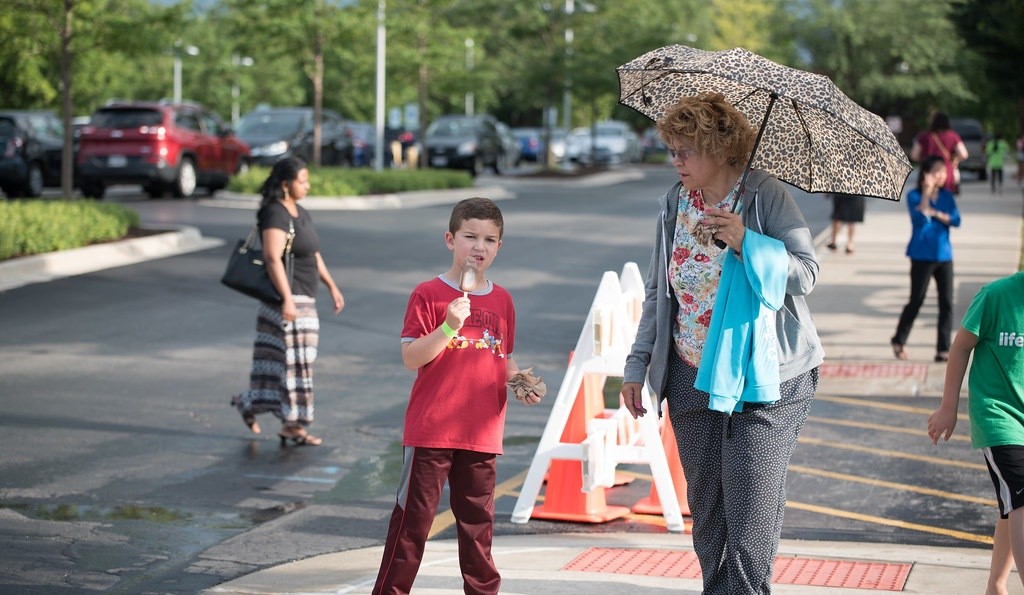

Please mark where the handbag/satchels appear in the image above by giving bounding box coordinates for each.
[953,169,960,185]
[221,219,295,306]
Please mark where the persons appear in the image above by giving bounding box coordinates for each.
[1015,134,1024,177]
[824,190,865,254]
[621,93,826,595]
[890,156,963,364]
[927,271,1024,595]
[231,156,345,445]
[909,108,969,194]
[985,132,1010,193]
[370,197,543,595]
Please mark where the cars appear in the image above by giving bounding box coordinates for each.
[348,124,414,170]
[1,110,78,201]
[511,129,589,169]
[572,122,668,170]
[415,115,508,176]
[236,107,352,169]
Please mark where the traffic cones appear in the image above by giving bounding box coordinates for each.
[632,397,692,517]
[529,348,632,525]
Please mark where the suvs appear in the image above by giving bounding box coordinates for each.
[950,120,987,181]
[77,99,250,198]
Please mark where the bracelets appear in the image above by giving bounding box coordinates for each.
[441,320,458,338]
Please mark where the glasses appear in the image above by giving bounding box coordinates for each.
[669,148,695,162]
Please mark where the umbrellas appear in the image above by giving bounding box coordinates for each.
[614,45,914,252]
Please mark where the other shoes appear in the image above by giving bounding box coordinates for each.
[846,247,853,253]
[827,243,836,249]
[891,338,907,360]
[935,355,948,362]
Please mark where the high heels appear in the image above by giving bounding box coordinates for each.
[278,427,322,447]
[230,395,261,435]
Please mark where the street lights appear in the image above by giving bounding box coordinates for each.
[232,58,252,120]
[172,43,200,104]
